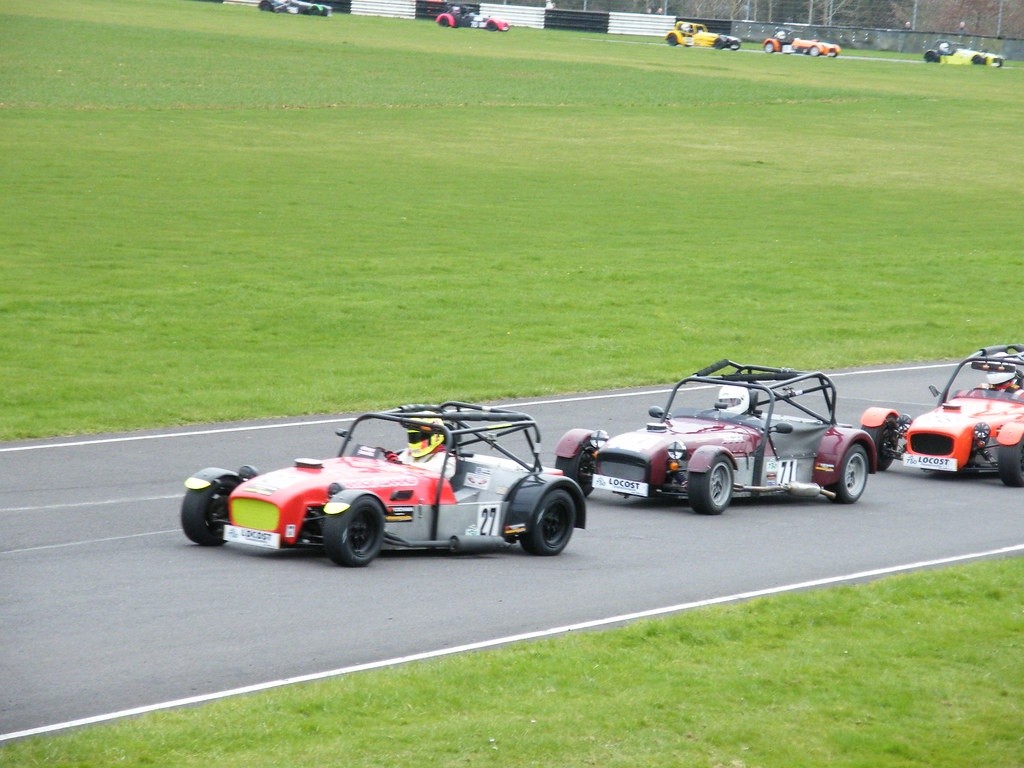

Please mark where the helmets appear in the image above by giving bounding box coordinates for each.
[987,351,1016,384]
[407,410,445,458]
[719,385,750,415]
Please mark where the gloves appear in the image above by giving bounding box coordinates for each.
[384,451,398,462]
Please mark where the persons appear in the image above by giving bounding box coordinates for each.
[384,410,456,482]
[718,385,760,430]
[680,23,692,34]
[957,351,1024,400]
[775,30,787,44]
[939,42,951,55]
